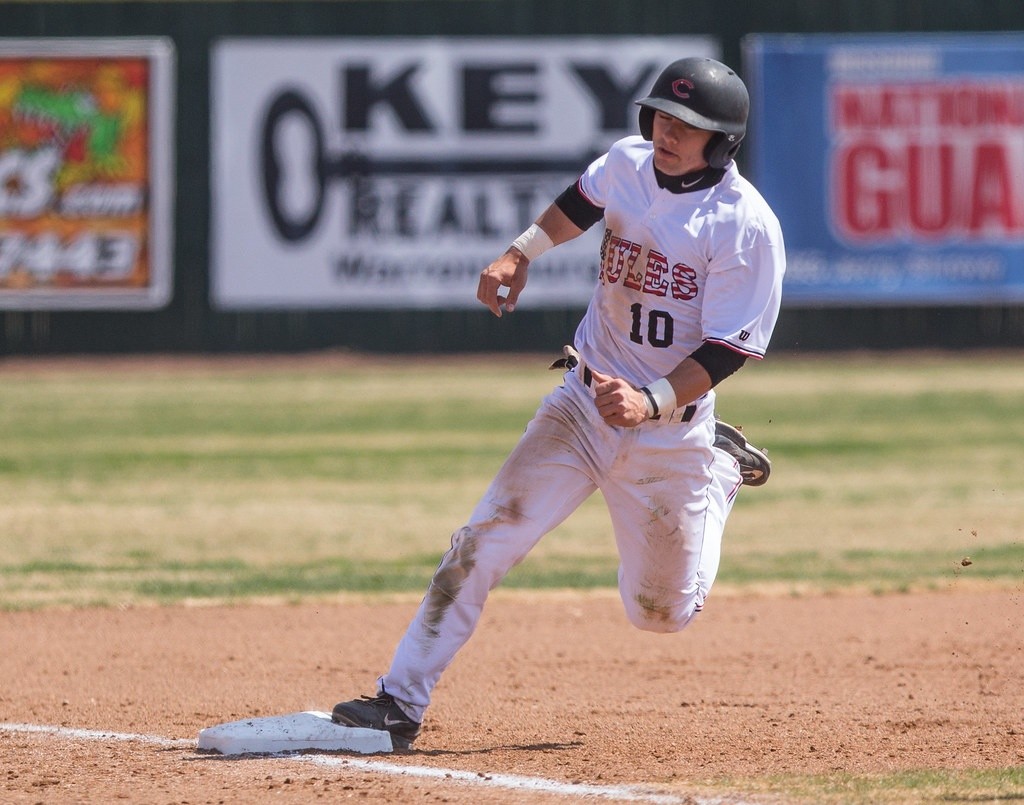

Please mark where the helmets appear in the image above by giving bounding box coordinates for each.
[634,57,749,168]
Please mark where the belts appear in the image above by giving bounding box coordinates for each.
[548,344,696,423]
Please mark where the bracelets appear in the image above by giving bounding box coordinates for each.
[511,222,554,261]
[641,378,677,418]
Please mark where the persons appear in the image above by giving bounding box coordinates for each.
[333,55,786,745]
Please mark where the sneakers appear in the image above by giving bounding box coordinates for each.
[332,677,421,749]
[714,413,771,486]
